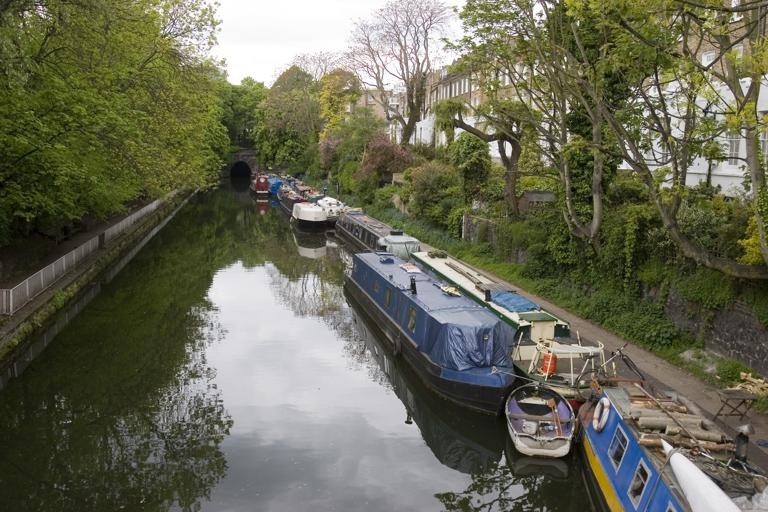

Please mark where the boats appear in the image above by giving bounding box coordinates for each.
[342,287,502,474]
[292,201,328,228]
[249,171,327,217]
[341,250,516,417]
[317,196,349,226]
[290,224,327,259]
[504,439,569,482]
[333,210,421,261]
[572,344,768,512]
[505,379,575,458]
[407,248,619,411]
